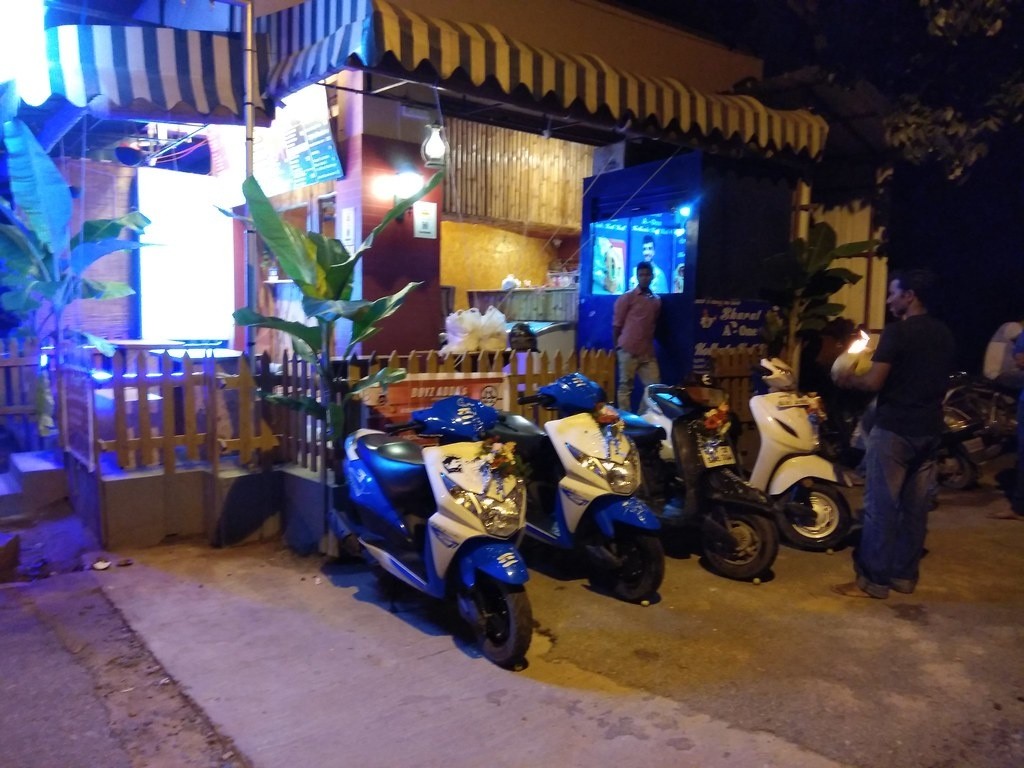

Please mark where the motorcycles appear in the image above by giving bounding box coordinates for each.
[725,354,856,552]
[331,391,539,670]
[637,374,785,579]
[820,374,976,492]
[516,369,670,606]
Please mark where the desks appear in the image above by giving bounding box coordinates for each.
[152,349,243,365]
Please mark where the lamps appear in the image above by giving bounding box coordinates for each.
[419,122,450,168]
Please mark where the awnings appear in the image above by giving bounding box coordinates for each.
[257,0,828,160]
[21,24,268,120]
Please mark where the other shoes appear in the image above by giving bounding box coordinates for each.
[885,576,916,593]
[831,580,874,597]
[986,509,1024,521]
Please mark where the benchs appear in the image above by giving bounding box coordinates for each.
[87,369,162,462]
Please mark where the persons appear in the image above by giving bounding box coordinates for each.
[983,322,1024,384]
[613,262,660,409]
[987,332,1024,518]
[833,270,951,598]
[631,236,667,293]
